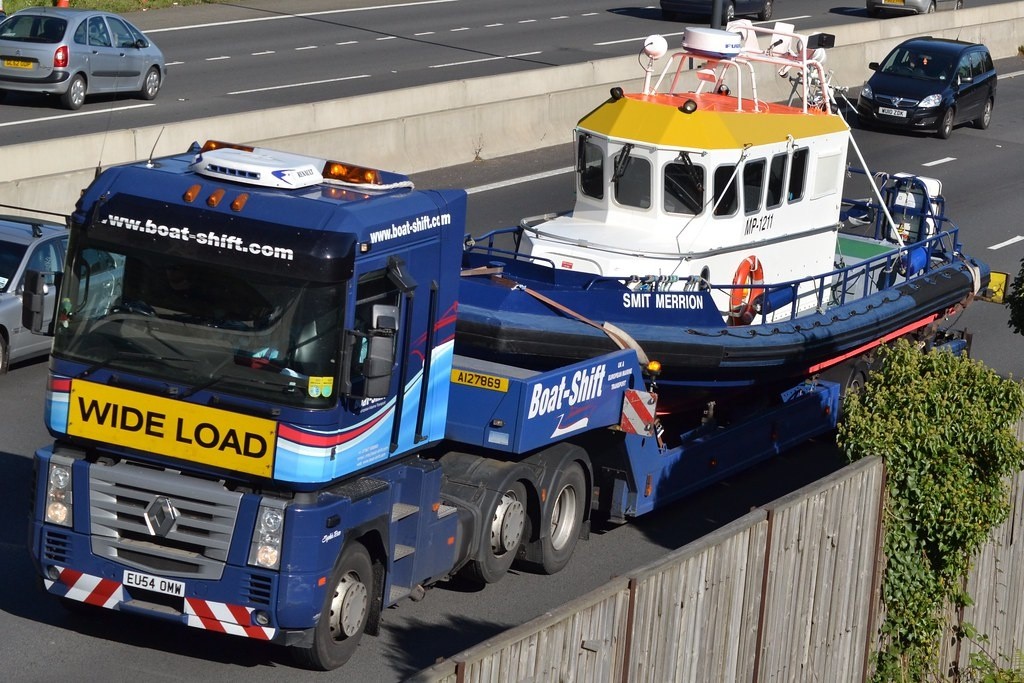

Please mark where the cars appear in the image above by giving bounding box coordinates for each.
[1,200,126,372]
[855,36,1001,137]
[655,0,774,30]
[862,0,962,17]
[0,7,170,111]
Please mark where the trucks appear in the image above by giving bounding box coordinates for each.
[26,42,1001,671]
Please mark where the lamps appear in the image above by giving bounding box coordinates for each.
[796,39,828,66]
[644,34,669,60]
